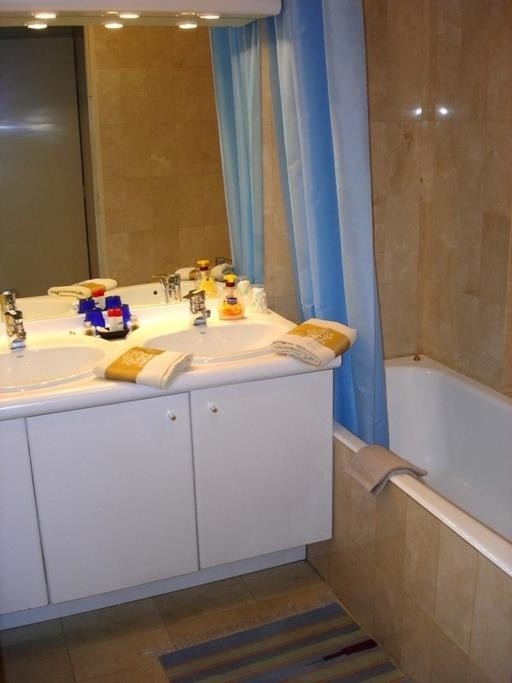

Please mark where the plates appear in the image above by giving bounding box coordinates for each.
[97,326,129,339]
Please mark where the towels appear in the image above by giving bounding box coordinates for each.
[270,318,357,369]
[343,444,427,495]
[174,264,235,280]
[48,277,118,300]
[91,346,192,389]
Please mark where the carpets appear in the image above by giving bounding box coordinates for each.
[161,600,411,682]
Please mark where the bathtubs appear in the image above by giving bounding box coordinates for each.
[332,354,511,578]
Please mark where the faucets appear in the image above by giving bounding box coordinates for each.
[4,308,27,350]
[0,288,17,321]
[181,288,211,327]
[151,272,181,304]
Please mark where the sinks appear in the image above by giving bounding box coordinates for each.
[105,279,222,306]
[0,296,83,320]
[0,344,109,393]
[141,321,288,362]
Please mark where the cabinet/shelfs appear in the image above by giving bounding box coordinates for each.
[2,415,52,629]
[25,367,335,622]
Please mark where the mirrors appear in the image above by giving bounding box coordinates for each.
[2,12,263,321]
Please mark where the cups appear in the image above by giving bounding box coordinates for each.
[115,304,132,329]
[104,295,121,309]
[77,298,96,314]
[85,306,105,335]
